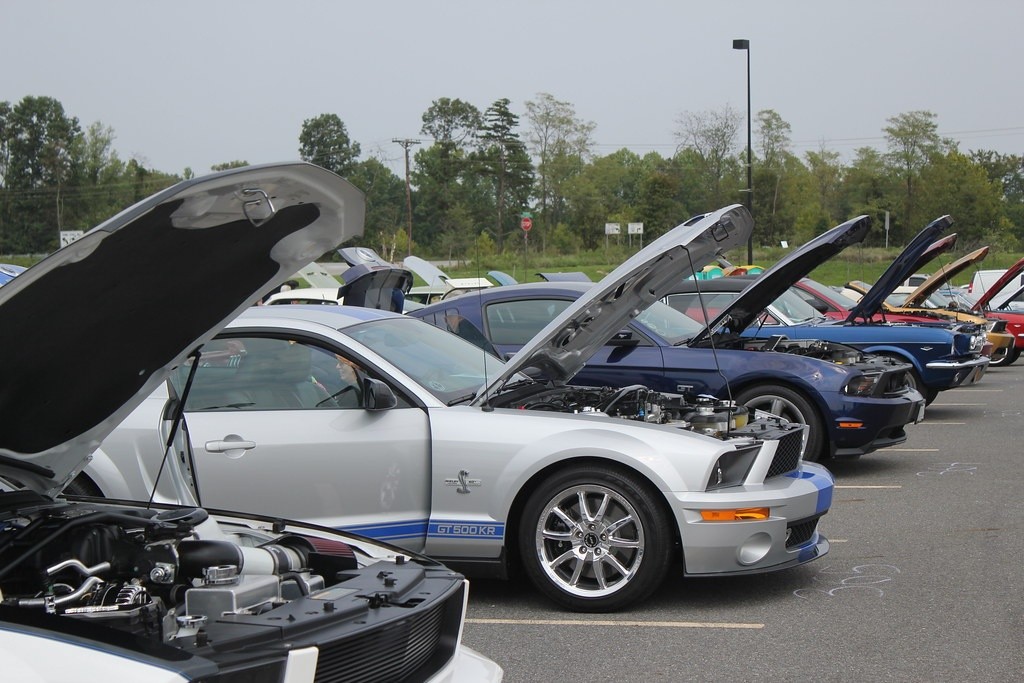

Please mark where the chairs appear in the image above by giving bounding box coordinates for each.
[218,360,279,409]
[268,344,338,408]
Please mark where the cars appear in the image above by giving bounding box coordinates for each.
[809,246,1015,372]
[60,203,836,614]
[3,159,504,683]
[398,212,927,471]
[790,231,993,395]
[846,254,1024,369]
[264,246,595,317]
[656,213,981,415]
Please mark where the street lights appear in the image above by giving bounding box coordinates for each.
[733,39,752,265]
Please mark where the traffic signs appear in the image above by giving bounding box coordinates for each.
[521,218,532,231]
